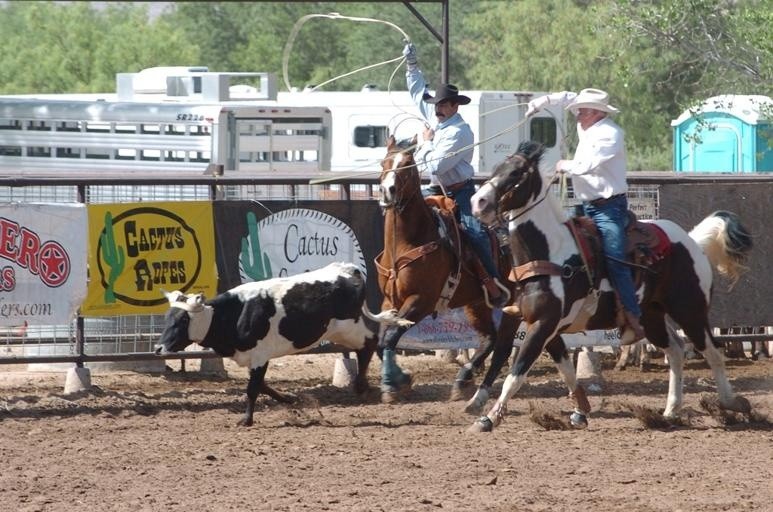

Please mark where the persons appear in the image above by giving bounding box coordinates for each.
[524,89,645,344]
[400,38,510,309]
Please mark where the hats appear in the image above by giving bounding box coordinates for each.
[423,84,471,104]
[566,88,620,116]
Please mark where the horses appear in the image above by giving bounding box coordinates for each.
[374,133,531,414]
[469,138,762,432]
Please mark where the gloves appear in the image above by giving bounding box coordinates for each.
[403,39,416,62]
[525,96,549,117]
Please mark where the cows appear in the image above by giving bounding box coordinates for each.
[154,261,416,427]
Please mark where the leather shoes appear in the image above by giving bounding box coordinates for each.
[620,317,639,344]
[489,279,504,307]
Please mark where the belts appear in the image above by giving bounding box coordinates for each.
[446,182,464,191]
[592,194,625,205]
